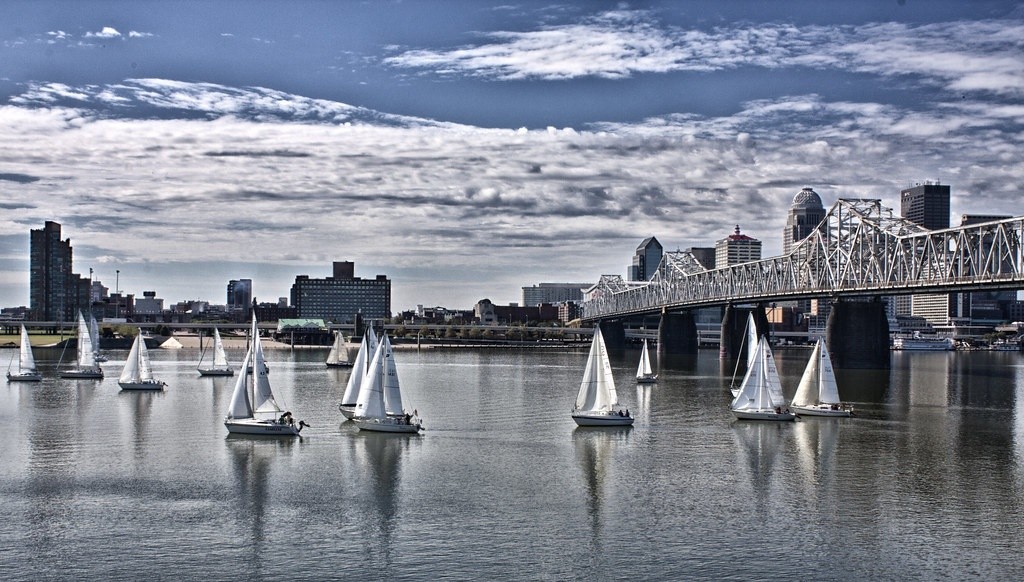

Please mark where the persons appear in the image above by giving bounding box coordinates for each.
[625,409,629,417]
[279,415,286,424]
[405,412,411,424]
[619,410,623,417]
[777,407,782,413]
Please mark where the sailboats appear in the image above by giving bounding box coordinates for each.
[571,323,635,425]
[118,328,169,391]
[789,336,854,417]
[6,324,42,381]
[196,327,235,376]
[339,320,425,433]
[326,333,353,367]
[55,309,104,378]
[636,337,659,382]
[223,309,310,435]
[729,312,796,420]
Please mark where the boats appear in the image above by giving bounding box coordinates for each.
[890,331,955,351]
[988,337,1019,351]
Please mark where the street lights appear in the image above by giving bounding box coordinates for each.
[117,270,120,318]
[509,314,511,327]
[526,315,528,327]
[89,267,94,309]
